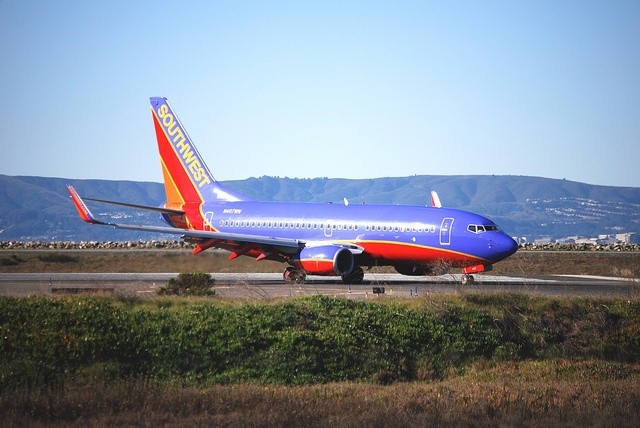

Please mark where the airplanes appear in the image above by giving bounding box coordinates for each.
[66,96,518,284]
